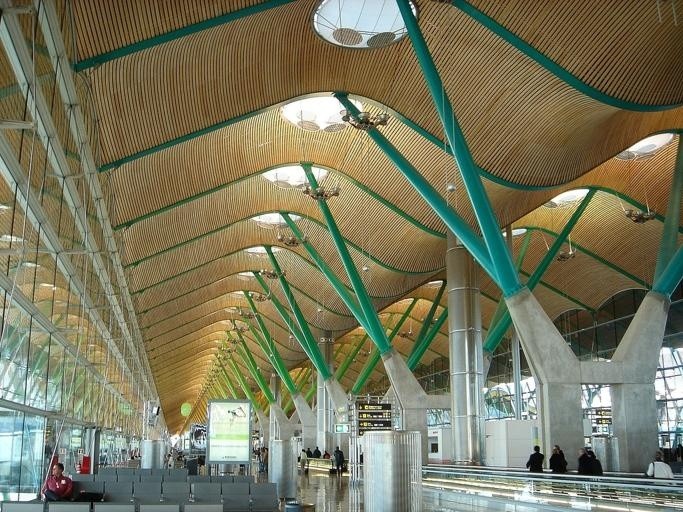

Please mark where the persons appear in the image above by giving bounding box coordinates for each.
[38,463,73,501]
[253,446,268,474]
[526,444,604,500]
[299,445,345,478]
[645,450,677,484]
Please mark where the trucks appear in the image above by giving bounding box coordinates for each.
[0,430,44,494]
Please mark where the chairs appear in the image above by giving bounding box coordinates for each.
[0,458,281,511]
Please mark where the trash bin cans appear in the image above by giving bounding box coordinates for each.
[240,464,245,475]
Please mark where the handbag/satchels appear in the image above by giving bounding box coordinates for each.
[70,490,105,502]
